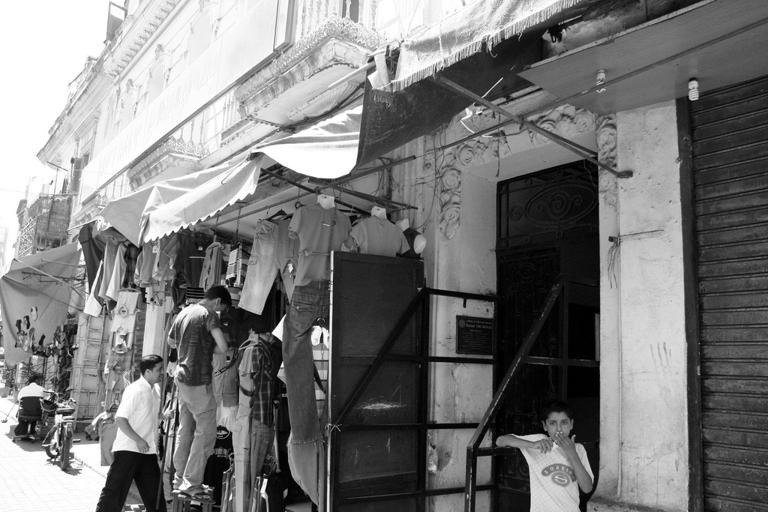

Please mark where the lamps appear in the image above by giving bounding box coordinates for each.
[687,79,700,101]
[594,69,608,93]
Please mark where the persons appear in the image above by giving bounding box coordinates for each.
[95,354,174,511]
[496,404,596,511]
[164,285,232,506]
[17,377,45,434]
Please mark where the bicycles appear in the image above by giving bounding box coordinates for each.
[41,388,81,470]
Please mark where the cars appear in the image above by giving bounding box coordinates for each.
[84,403,116,440]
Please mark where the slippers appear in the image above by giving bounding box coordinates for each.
[173,474,217,505]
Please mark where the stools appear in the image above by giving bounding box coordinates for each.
[171,486,215,511]
